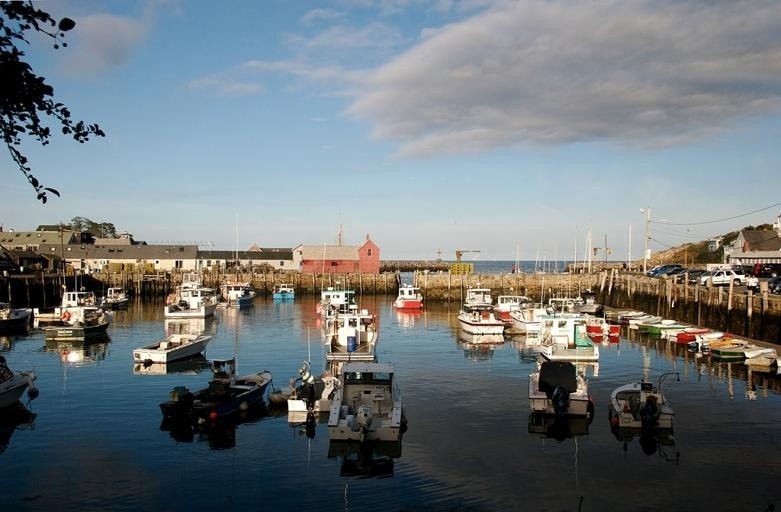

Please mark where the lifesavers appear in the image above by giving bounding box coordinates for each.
[317,303,323,313]
[61,312,71,321]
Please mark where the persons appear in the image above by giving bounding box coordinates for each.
[720,272,724,276]
[512,263,517,274]
[746,272,760,295]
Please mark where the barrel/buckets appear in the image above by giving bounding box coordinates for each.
[347,336,356,352]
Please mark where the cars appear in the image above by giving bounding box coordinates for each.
[646,264,781,295]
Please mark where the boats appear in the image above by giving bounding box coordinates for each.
[393,283,424,309]
[0,285,128,406]
